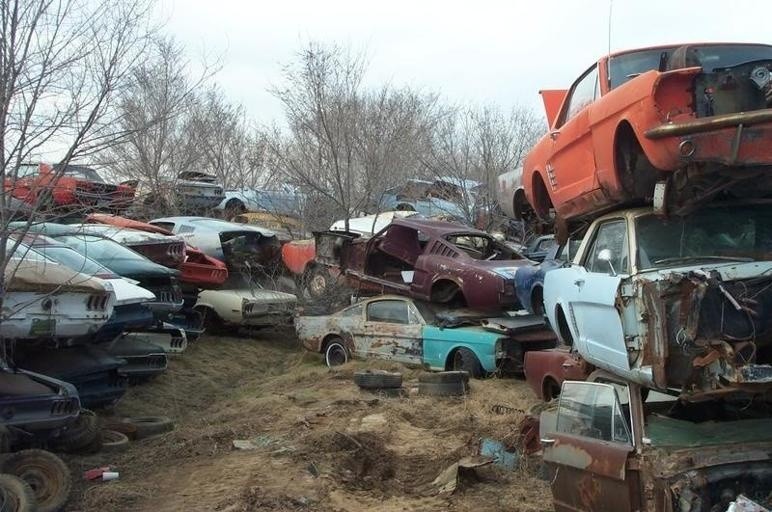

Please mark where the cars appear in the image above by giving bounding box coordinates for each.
[517,44,772,230]
[0,155,496,455]
[535,366,772,512]
[539,200,771,403]
[293,293,558,380]
[312,216,539,314]
